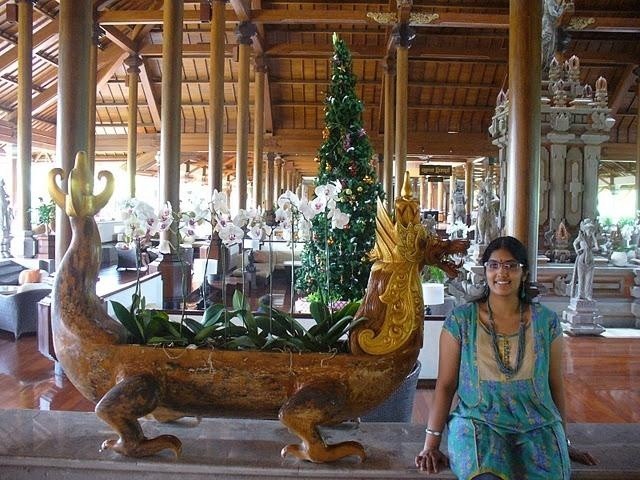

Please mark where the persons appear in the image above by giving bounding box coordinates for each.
[572,221,600,301]
[413,234,601,479]
[476,182,501,244]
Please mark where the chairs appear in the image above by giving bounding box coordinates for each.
[0,282,53,341]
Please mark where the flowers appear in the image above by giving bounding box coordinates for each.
[107,185,371,356]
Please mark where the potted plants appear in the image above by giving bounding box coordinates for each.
[27,200,61,260]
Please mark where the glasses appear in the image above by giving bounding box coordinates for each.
[484,260,524,271]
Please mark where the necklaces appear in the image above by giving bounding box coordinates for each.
[487,298,526,376]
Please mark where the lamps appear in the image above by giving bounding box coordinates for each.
[419,283,446,315]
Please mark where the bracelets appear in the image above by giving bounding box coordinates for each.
[426,428,444,437]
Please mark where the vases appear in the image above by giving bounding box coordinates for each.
[362,358,423,424]
